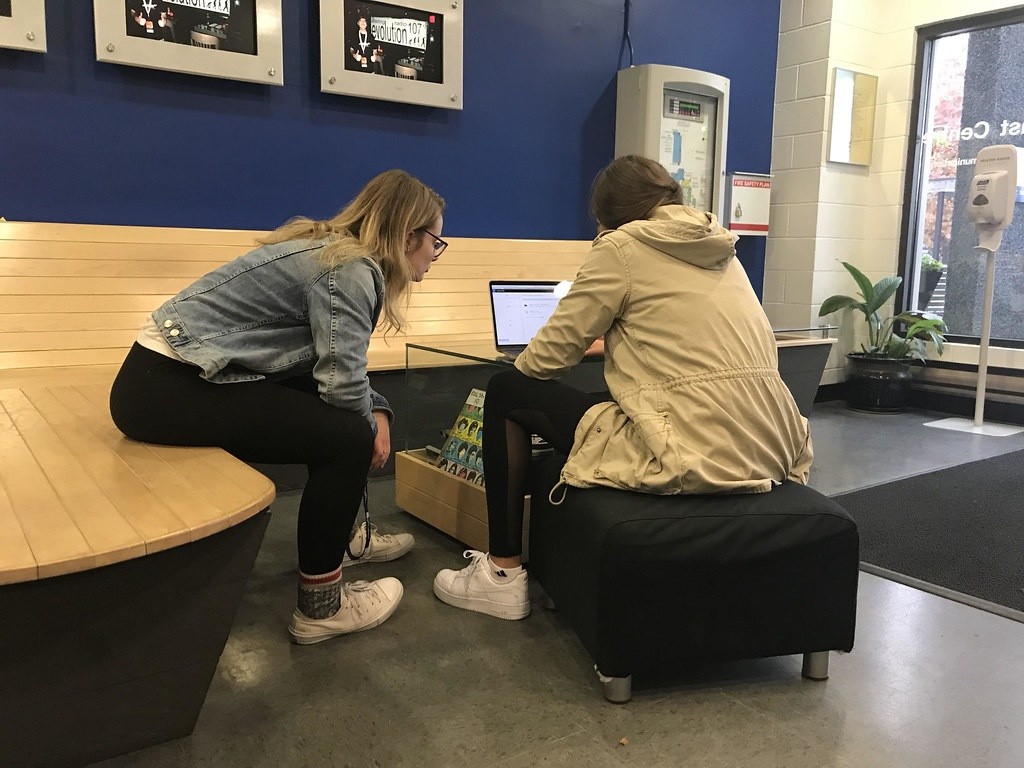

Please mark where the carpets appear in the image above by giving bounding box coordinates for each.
[826,447,1024,625]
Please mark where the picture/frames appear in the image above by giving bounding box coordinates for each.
[0,0,48,54]
[319,0,464,110]
[93,0,284,87]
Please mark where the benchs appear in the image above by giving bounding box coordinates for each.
[0,218,610,768]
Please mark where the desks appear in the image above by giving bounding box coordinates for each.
[394,323,839,564]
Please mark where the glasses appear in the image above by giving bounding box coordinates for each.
[420,227,448,257]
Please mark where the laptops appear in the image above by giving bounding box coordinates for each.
[489,280,605,363]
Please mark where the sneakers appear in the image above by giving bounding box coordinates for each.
[343,522,414,568]
[433,549,531,620]
[287,577,404,645]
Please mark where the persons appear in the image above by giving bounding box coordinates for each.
[431,153,816,623]
[349,15,377,74]
[106,168,448,648]
[130,0,163,41]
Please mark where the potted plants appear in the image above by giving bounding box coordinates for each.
[918,253,946,317]
[818,261,949,415]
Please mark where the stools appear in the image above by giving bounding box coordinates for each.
[528,451,861,706]
